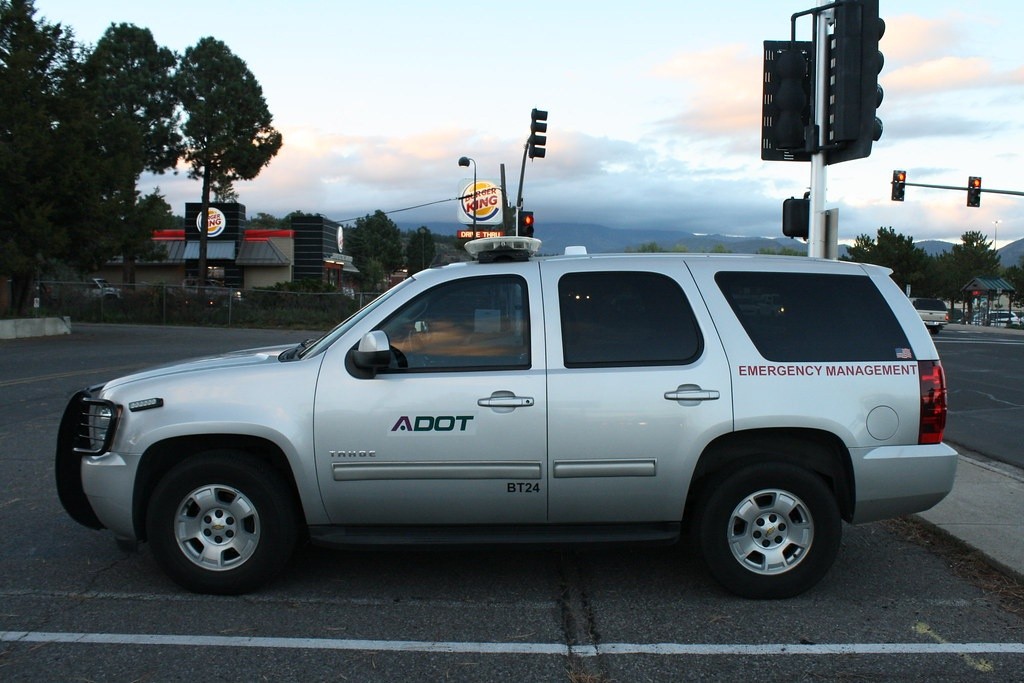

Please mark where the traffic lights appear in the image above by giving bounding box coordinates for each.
[967,176,982,207]
[829,0,887,165]
[518,211,534,238]
[529,108,548,157]
[891,170,906,201]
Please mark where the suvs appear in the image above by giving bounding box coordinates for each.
[56,236,958,600]
[908,298,949,334]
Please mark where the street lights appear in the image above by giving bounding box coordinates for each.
[459,157,477,240]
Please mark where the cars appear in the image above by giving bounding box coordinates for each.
[948,309,1024,327]
[182,278,242,305]
[82,278,122,301]
